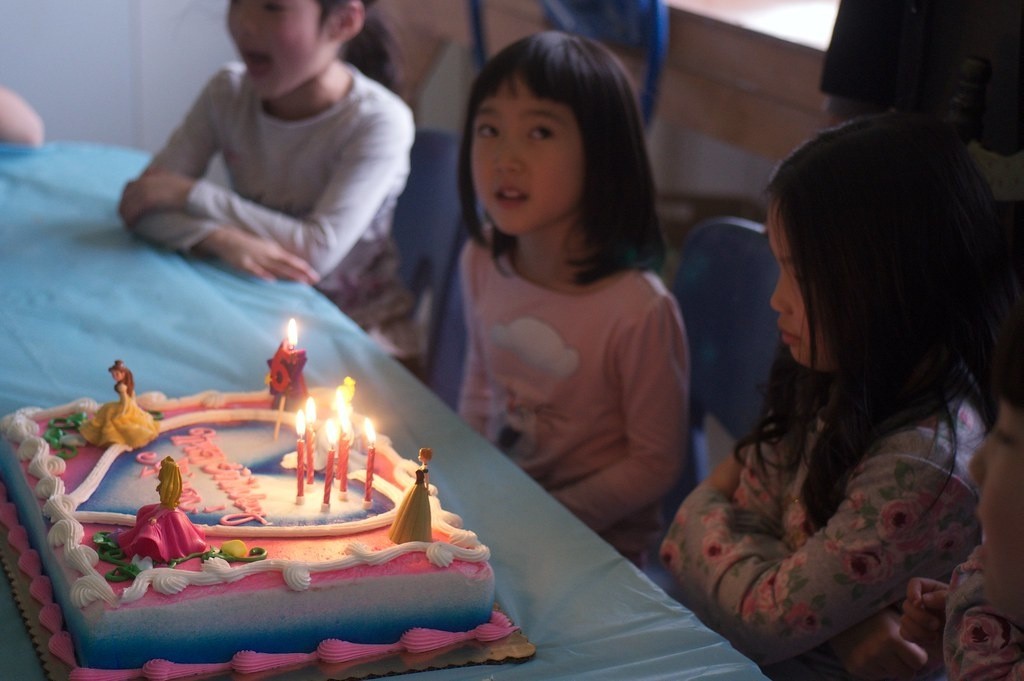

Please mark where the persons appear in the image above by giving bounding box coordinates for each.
[657,111,1013,681]
[897,335,1024,681]
[116,0,427,383]
[455,29,690,565]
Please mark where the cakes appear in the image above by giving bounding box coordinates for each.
[0,388,518,680]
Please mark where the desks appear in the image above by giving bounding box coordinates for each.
[0,142,769,680]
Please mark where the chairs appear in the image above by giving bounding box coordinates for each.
[400,129,467,407]
[624,212,780,603]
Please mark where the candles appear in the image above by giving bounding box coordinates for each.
[263,320,381,513]
